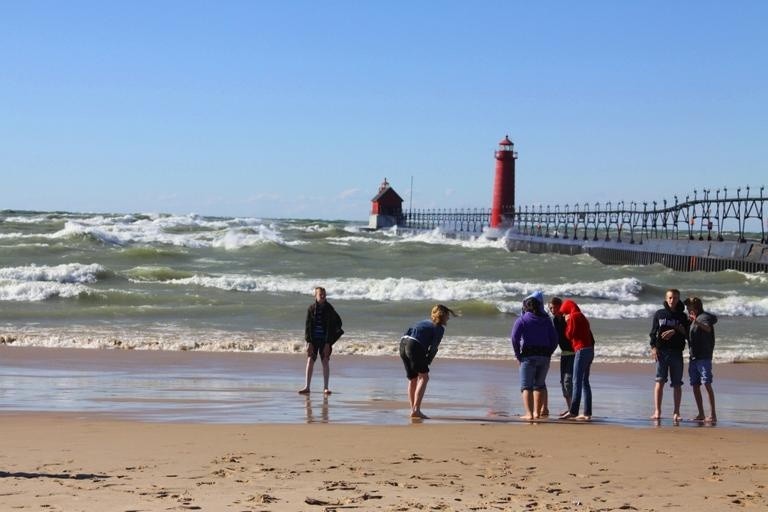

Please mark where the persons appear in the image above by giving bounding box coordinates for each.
[699,420,716,427]
[520,292,550,415]
[398,304,463,420]
[303,394,329,423]
[299,287,345,394]
[512,297,557,421]
[649,289,688,420]
[548,297,576,416]
[677,298,718,422]
[558,299,595,421]
[654,419,679,426]
